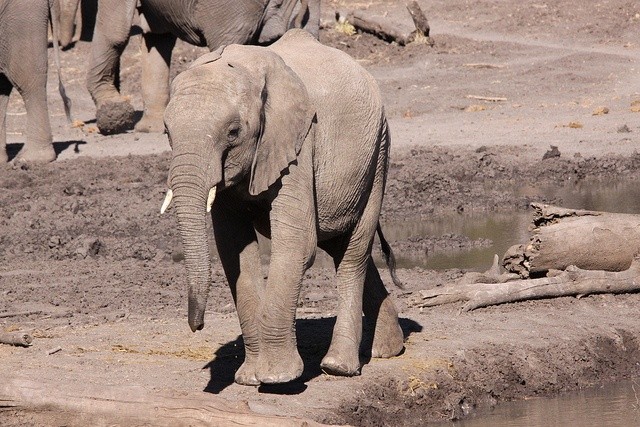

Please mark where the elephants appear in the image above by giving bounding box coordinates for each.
[158,27,405,387]
[58,0,321,135]
[0,0,73,166]
